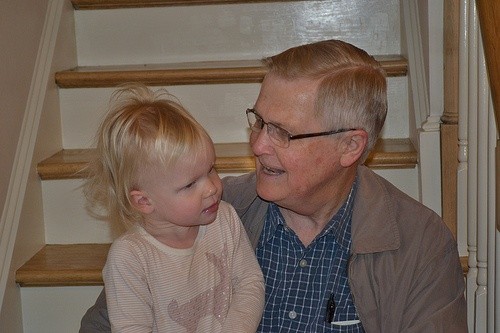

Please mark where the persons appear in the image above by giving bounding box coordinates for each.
[78,40,468,333]
[74,82,266,333]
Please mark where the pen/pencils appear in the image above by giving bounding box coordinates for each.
[326,293,336,323]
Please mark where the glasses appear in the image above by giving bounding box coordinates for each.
[245,107,360,149]
